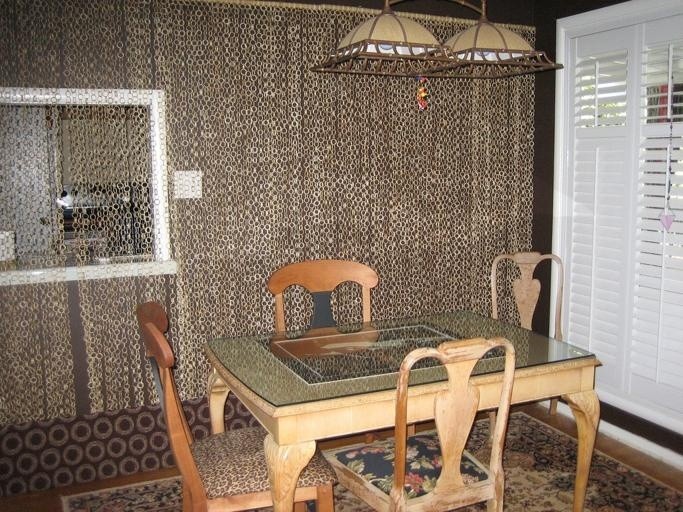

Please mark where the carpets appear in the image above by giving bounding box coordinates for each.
[60,410,683,511]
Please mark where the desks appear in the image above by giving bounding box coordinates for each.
[202,309,601,509]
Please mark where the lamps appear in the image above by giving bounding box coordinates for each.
[308,0,564,79]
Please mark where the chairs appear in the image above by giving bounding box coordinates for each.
[265,259,416,450]
[143,322,336,510]
[325,338,517,510]
[488,252,564,418]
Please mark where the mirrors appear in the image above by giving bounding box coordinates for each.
[0,88,184,287]
